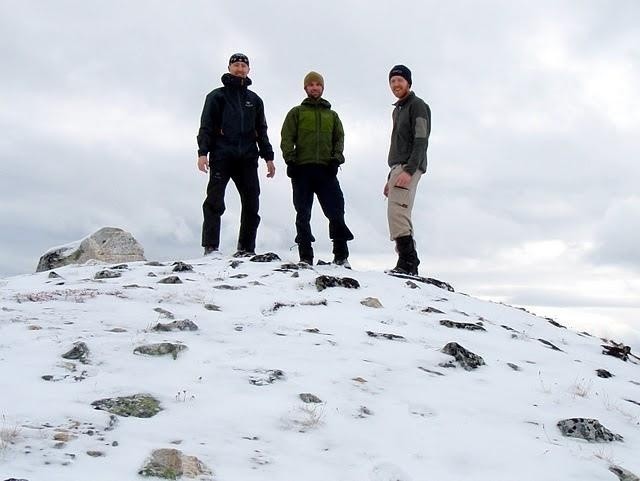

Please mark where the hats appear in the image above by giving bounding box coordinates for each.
[229,52,249,66]
[389,65,412,84]
[304,71,324,88]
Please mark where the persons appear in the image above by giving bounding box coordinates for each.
[196,53,276,257]
[280,72,354,268]
[383,65,432,276]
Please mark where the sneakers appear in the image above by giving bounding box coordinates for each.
[390,265,418,276]
[298,258,313,267]
[333,256,351,269]
